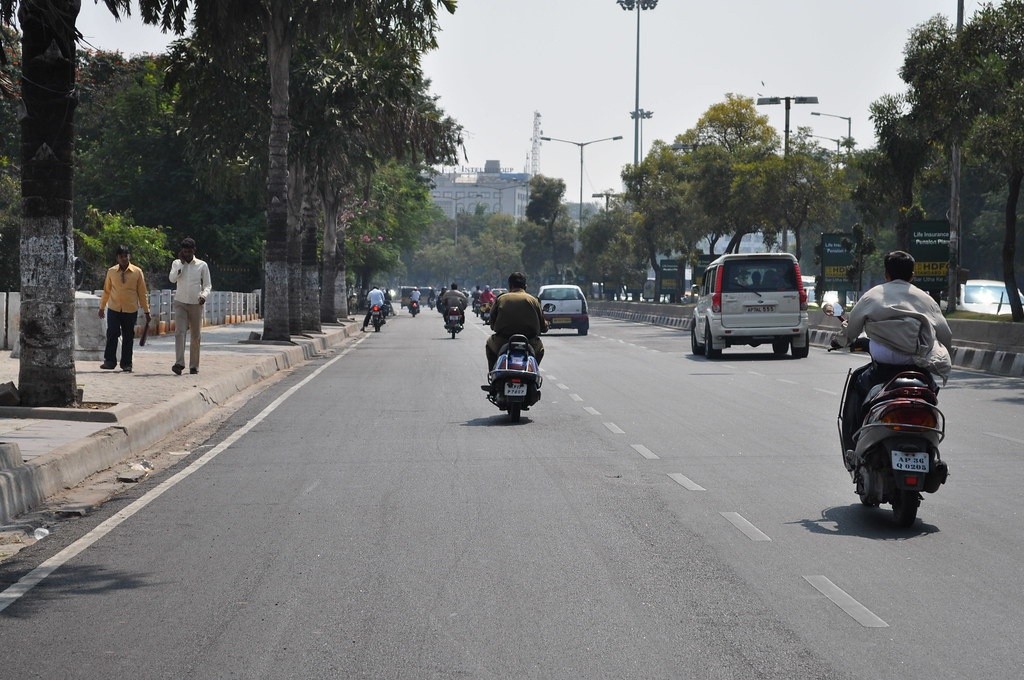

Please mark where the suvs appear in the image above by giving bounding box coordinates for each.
[400,286,434,309]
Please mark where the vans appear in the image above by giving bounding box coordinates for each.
[689,252,810,360]
[929,279,1024,317]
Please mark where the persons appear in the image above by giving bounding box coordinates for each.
[822,303,835,315]
[471,286,482,312]
[360,285,388,332]
[486,271,549,380]
[481,285,495,305]
[97,245,152,373]
[428,289,436,301]
[439,285,448,297]
[683,295,689,302]
[461,287,469,299]
[408,286,422,313]
[829,250,953,455]
[442,281,468,325]
[169,237,213,376]
[750,271,762,285]
[383,289,397,316]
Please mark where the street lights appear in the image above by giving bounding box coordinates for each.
[473,184,526,213]
[806,134,840,168]
[811,112,851,152]
[432,195,483,247]
[541,135,624,224]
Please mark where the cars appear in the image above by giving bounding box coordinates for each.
[490,288,509,296]
[803,286,853,306]
[537,284,590,336]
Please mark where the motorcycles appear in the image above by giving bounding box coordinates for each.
[370,297,490,340]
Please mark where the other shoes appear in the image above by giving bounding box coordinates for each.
[100,360,117,369]
[172,366,181,375]
[123,366,132,371]
[190,368,197,374]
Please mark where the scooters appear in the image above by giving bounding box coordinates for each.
[819,299,949,535]
[480,335,543,426]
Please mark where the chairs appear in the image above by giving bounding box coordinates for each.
[761,269,775,286]
